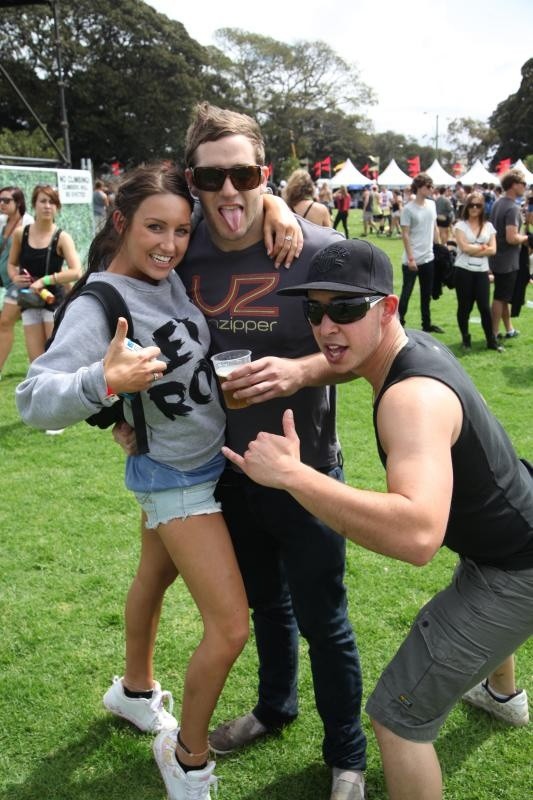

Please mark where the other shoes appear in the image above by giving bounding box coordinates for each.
[423,326,443,333]
[506,330,520,338]
[330,765,365,800]
[207,712,268,755]
[463,344,471,352]
[487,342,505,353]
[494,333,504,339]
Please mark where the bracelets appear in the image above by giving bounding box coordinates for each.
[53,272,57,285]
[44,275,50,286]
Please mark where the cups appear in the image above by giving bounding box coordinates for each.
[210,351,254,410]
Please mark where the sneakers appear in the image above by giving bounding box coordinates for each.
[462,678,529,726]
[153,726,217,800]
[102,674,177,735]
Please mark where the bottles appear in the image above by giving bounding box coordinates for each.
[35,287,56,304]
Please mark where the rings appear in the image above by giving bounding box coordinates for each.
[153,372,158,381]
[285,236,292,241]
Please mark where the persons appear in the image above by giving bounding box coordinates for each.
[0,168,533,436]
[221,235,533,800]
[17,167,304,800]
[111,100,368,800]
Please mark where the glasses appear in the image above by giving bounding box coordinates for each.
[190,165,263,192]
[425,183,434,189]
[0,198,15,204]
[468,202,484,209]
[302,292,387,326]
[519,181,526,186]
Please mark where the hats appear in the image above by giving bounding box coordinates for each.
[275,238,394,298]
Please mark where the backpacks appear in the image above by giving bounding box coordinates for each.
[45,280,151,455]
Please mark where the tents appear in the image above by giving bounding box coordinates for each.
[328,158,533,187]
